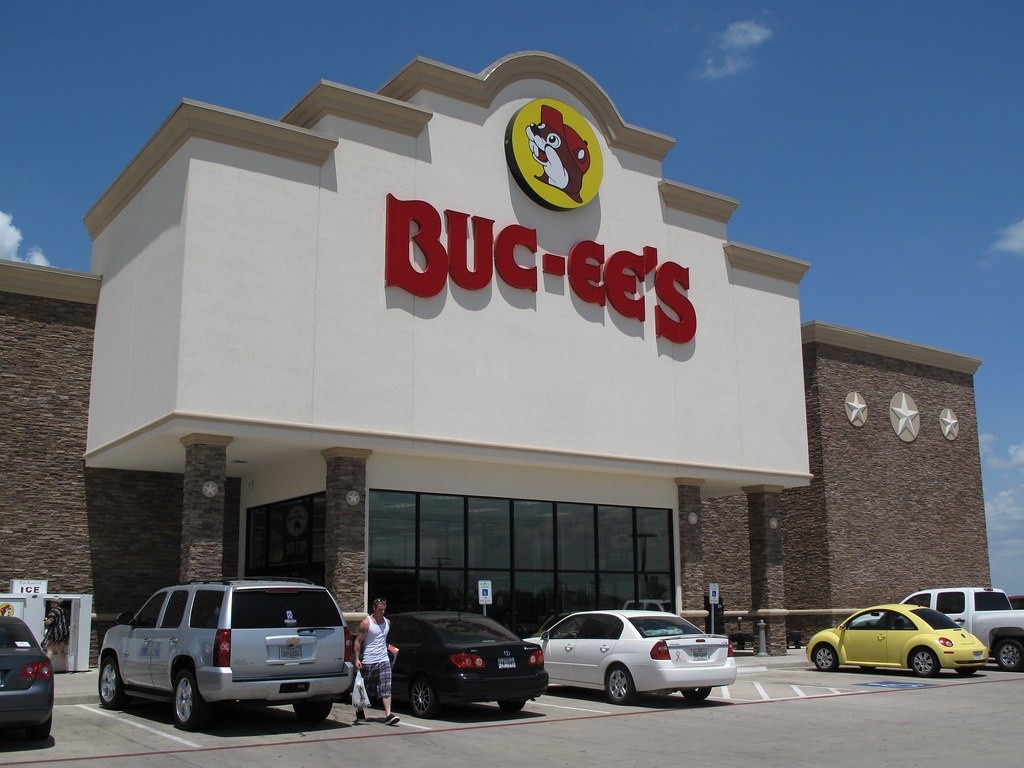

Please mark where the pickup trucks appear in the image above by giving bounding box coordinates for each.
[896,585,1024,671]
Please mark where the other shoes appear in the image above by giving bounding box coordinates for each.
[356,709,366,720]
[386,715,400,726]
[55,670,66,674]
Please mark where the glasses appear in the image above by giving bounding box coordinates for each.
[374,598,387,605]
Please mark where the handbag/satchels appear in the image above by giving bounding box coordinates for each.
[41,639,52,650]
[351,669,372,709]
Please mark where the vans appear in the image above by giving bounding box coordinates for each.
[623,599,672,612]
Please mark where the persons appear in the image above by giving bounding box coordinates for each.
[41,597,70,672]
[351,597,401,726]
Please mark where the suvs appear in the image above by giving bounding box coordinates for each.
[97,577,355,732]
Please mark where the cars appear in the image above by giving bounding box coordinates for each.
[531,611,582,639]
[806,603,990,679]
[1006,594,1024,610]
[522,608,739,706]
[341,608,550,720]
[0,615,54,738]
